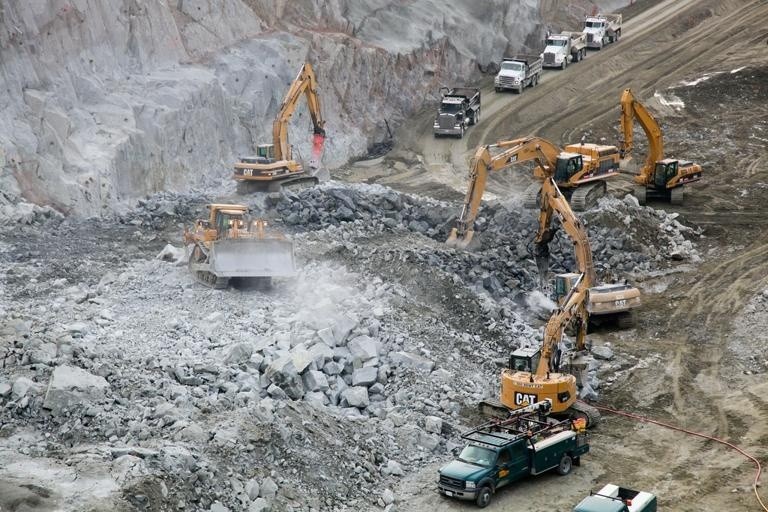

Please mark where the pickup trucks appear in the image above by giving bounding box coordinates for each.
[436,397,657,512]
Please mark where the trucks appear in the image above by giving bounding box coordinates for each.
[433,11,623,138]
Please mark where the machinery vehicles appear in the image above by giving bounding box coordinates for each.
[186,61,332,290]
